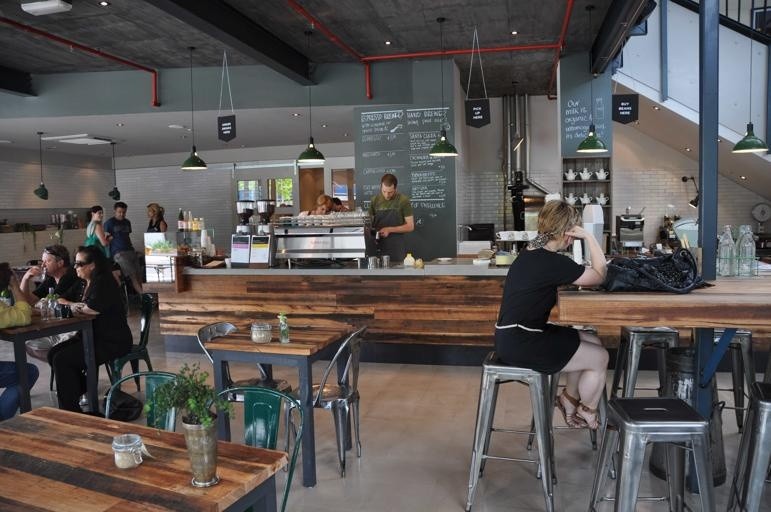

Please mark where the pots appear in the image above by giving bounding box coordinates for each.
[50,214,72,228]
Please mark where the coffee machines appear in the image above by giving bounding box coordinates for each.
[250,199,277,268]
[231,200,254,268]
[616,213,646,255]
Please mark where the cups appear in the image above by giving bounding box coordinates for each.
[368,255,391,267]
[279,210,369,227]
[54,301,64,318]
[201,230,216,256]
[41,302,50,321]
[656,244,663,250]
[216,248,225,256]
[34,268,46,283]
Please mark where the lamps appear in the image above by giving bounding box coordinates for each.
[176,44,211,173]
[107,138,121,203]
[32,130,49,201]
[427,13,460,159]
[729,1,771,154]
[574,4,607,154]
[19,0,72,16]
[681,175,699,209]
[295,26,328,165]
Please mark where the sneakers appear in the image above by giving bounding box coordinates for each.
[138,292,157,309]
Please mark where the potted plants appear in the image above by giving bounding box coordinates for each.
[277,312,289,344]
[142,361,249,487]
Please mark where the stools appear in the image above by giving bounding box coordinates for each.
[589,397,716,512]
[465,342,554,511]
[525,327,771,451]
[726,382,771,512]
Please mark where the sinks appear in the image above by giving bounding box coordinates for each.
[457,241,492,255]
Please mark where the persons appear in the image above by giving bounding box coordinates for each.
[0,262,39,421]
[146,203,167,232]
[330,198,348,212]
[297,195,332,227]
[366,174,415,261]
[493,200,608,429]
[49,244,134,410]
[104,202,144,296]
[85,206,113,258]
[20,245,86,361]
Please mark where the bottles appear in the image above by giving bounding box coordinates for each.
[112,434,144,469]
[178,207,206,232]
[404,253,416,265]
[719,224,756,276]
[48,288,58,319]
[72,214,79,229]
[669,221,676,239]
[279,311,289,343]
[627,206,632,215]
[376,232,382,240]
[277,199,294,207]
[250,321,272,344]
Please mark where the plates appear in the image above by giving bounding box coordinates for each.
[437,257,453,261]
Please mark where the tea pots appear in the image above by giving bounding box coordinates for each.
[595,168,610,180]
[595,193,610,205]
[565,193,578,206]
[565,169,578,180]
[579,193,593,205]
[580,168,593,180]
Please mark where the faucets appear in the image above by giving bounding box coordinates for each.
[458,224,473,241]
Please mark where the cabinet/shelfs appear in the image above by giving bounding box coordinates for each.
[562,152,615,256]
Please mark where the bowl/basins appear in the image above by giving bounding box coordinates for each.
[16,222,47,231]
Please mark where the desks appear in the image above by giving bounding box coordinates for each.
[1,406,291,512]
[203,319,357,488]
[1,309,100,417]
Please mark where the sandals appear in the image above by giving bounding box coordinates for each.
[574,402,600,429]
[556,388,583,429]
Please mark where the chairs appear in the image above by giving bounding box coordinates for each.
[105,371,194,434]
[202,385,305,512]
[197,321,292,472]
[282,321,368,479]
[49,293,155,391]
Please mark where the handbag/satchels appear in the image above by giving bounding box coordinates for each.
[606,247,701,293]
[84,226,108,256]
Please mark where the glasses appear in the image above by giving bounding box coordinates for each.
[74,259,94,268]
